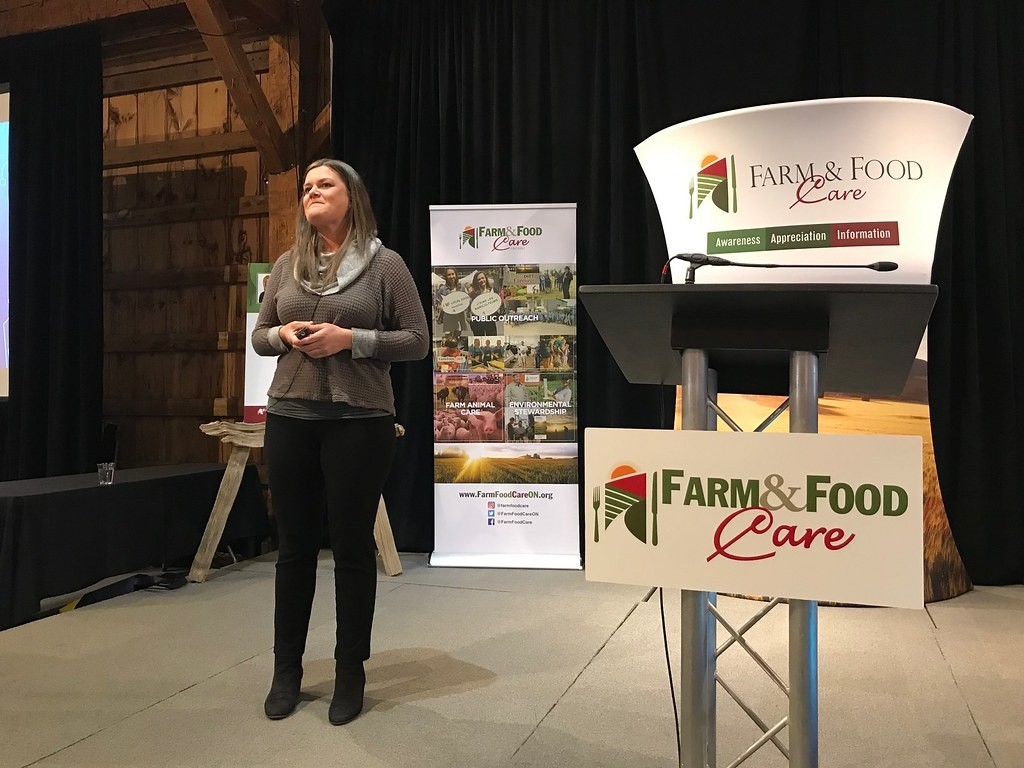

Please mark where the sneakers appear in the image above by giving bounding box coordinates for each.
[263,655,303,721]
[328,658,365,723]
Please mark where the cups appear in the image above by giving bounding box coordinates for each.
[97,462,116,484]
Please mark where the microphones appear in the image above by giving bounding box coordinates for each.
[675,254,899,272]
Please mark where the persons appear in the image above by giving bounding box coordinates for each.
[432,264,576,441]
[251,160,430,724]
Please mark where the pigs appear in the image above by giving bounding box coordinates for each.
[467,408,502,440]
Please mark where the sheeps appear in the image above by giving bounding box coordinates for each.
[433,409,473,440]
